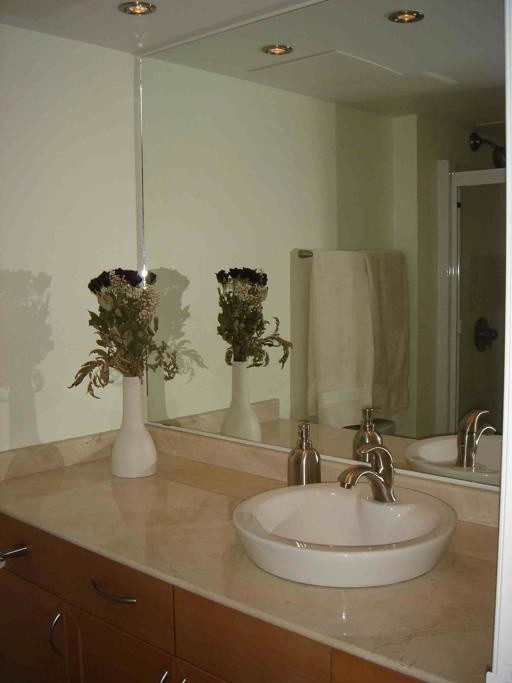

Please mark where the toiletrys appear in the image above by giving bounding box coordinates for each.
[288,422,321,485]
[352,407,384,463]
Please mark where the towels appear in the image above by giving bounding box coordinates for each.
[303,248,414,429]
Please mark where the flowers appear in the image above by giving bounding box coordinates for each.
[215,265,295,369]
[63,263,177,405]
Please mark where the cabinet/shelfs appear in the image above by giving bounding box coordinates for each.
[63,541,333,682]
[2,515,68,682]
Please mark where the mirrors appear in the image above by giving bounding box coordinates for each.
[133,4,512,494]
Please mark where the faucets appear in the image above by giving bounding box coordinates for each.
[457,407,496,467]
[338,444,397,503]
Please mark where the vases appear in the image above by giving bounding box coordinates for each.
[222,357,263,445]
[110,376,159,480]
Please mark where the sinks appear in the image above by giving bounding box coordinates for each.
[234,483,457,588]
[404,433,502,487]
